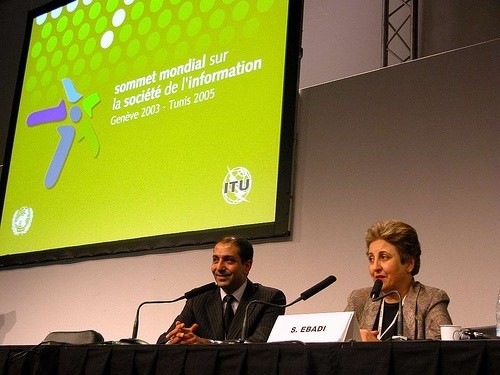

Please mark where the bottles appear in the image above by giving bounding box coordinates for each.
[496,290,500,337]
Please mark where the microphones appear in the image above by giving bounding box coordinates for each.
[236,275,337,343]
[118,281,217,346]
[370,279,411,341]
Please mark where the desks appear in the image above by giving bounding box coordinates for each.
[0,338,500,375]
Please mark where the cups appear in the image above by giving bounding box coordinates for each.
[440,325,461,340]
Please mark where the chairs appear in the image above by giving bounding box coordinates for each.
[42,330,103,345]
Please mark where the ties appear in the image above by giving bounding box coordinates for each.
[222,294,236,336]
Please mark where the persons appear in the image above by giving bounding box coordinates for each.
[156,235,287,347]
[342,219,453,341]
